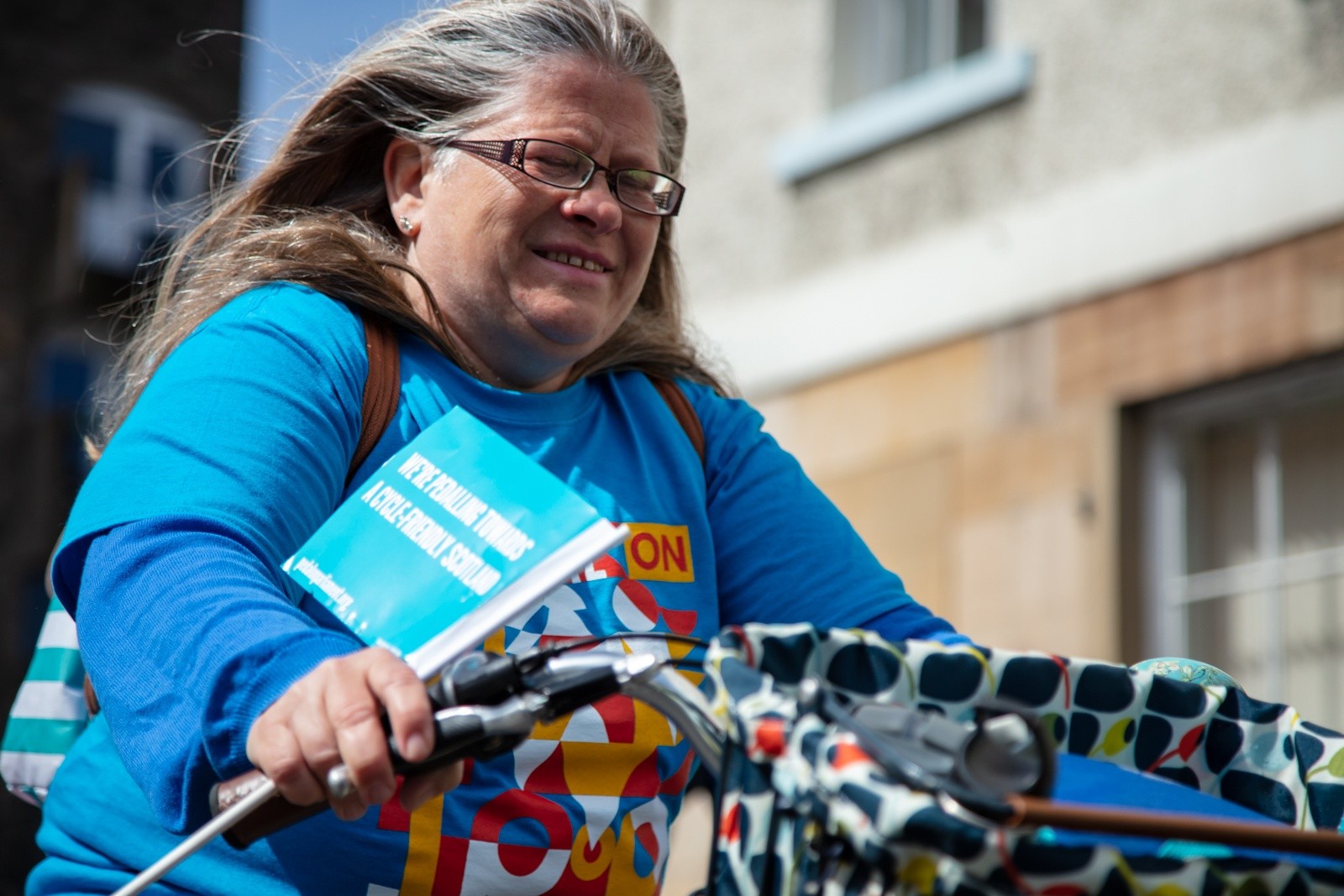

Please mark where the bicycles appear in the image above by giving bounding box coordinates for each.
[208,620,1231,896]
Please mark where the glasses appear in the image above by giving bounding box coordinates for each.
[441,138,686,219]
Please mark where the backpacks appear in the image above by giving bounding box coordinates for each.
[1,299,707,813]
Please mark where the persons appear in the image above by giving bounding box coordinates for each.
[29,1,988,896]
